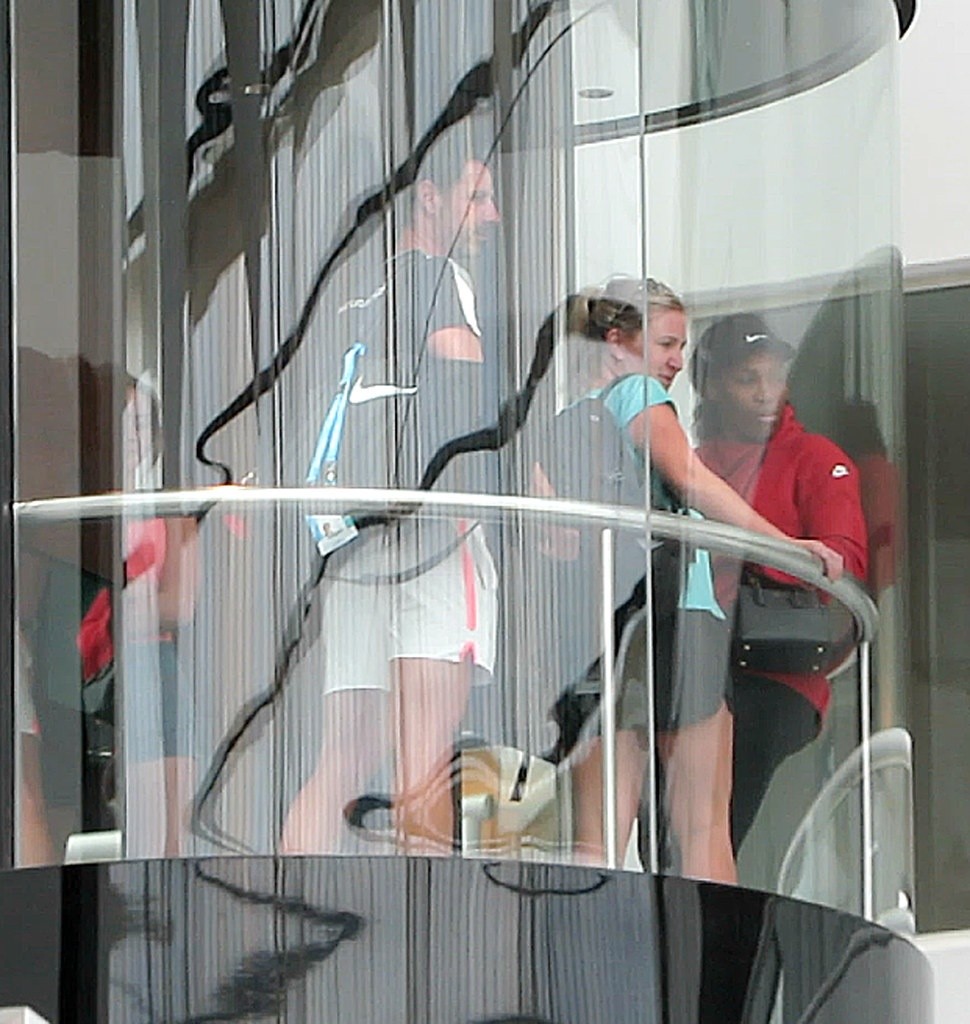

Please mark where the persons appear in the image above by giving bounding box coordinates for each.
[16,344,202,867]
[638,314,871,873]
[276,143,581,856]
[527,277,843,887]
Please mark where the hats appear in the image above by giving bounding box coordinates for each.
[690,312,794,393]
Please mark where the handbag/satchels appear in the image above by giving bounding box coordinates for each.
[731,563,828,676]
[534,368,644,512]
